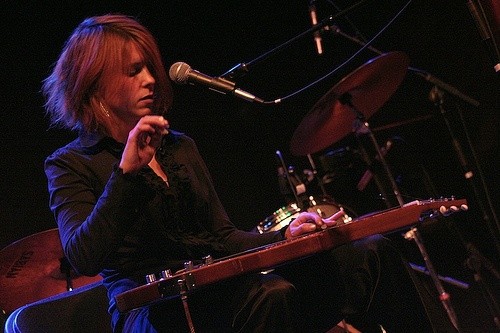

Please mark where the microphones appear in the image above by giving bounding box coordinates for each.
[169,62,264,105]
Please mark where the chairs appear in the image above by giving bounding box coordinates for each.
[4,281,113,333]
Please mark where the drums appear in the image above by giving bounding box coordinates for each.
[255,195,353,235]
[275,146,359,203]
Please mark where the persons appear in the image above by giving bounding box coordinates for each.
[41,13,347,333]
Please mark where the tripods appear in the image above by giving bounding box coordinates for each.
[422,171,500,333]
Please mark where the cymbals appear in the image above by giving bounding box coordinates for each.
[0,229,105,313]
[288,49,409,156]
